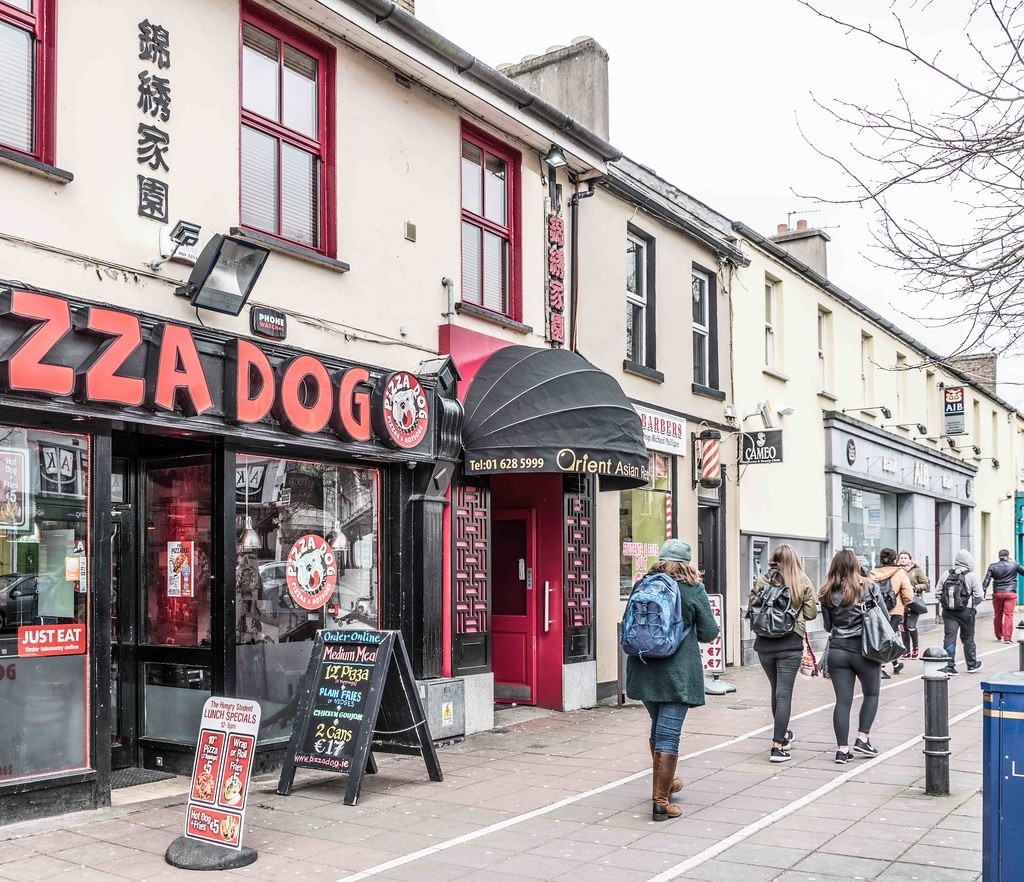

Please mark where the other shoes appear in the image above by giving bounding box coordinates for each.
[997,637,1002,640]
[1004,640,1012,644]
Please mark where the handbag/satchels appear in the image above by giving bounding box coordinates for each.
[906,591,928,613]
[817,639,831,679]
[862,584,908,664]
[797,631,818,677]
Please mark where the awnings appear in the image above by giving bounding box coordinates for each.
[459,345,648,492]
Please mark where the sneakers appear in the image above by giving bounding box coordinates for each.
[881,670,891,680]
[911,649,920,660]
[770,747,792,761]
[966,661,983,672]
[948,666,959,675]
[899,651,911,659]
[835,750,855,763]
[894,663,904,674]
[853,737,879,755]
[782,731,795,750]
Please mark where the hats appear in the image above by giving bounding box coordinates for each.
[659,538,692,563]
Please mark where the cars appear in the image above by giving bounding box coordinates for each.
[0,571,88,633]
[235,557,370,628]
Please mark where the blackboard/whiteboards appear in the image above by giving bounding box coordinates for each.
[286,629,443,772]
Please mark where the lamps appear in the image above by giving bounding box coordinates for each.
[962,457,1000,467]
[777,406,794,416]
[913,435,955,447]
[236,457,262,548]
[691,429,723,491]
[940,445,981,455]
[881,423,927,435]
[150,221,202,269]
[172,234,273,317]
[543,144,568,169]
[843,406,891,419]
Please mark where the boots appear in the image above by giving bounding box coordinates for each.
[653,750,682,822]
[649,738,683,802]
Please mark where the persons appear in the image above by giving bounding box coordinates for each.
[871,548,913,680]
[626,537,719,821]
[982,550,1024,643]
[897,551,930,660]
[818,550,892,764]
[935,549,983,676]
[753,544,818,762]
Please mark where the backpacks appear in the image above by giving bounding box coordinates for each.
[941,569,972,612]
[748,571,803,638]
[620,574,692,665]
[872,567,903,611]
